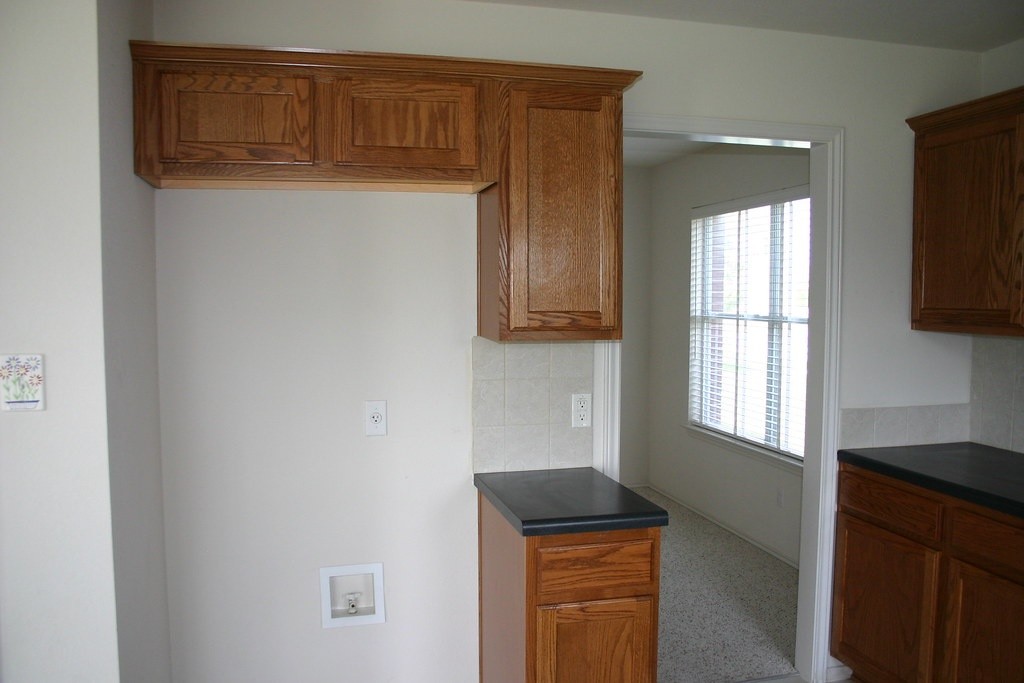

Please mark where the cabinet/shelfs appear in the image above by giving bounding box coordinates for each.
[905,85,1024,337]
[828,442,1024,683]
[127,40,643,345]
[473,468,667,683]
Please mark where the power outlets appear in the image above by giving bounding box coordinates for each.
[365,400,387,438]
[571,393,592,429]
